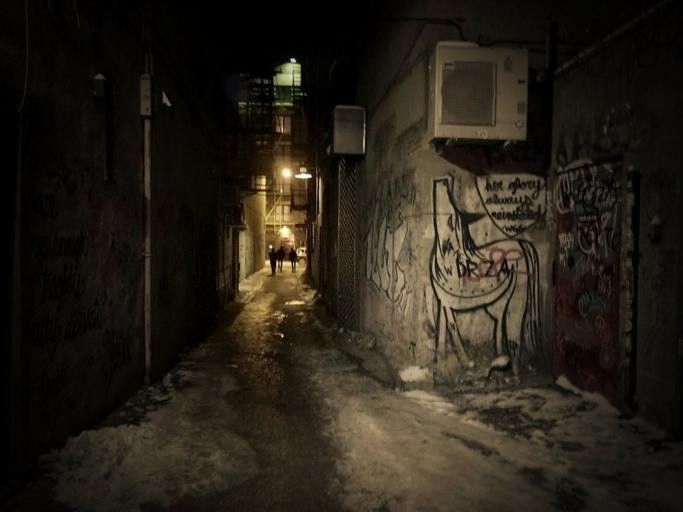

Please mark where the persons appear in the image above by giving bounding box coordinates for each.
[288,247,298,273]
[268,248,279,276]
[276,245,285,273]
